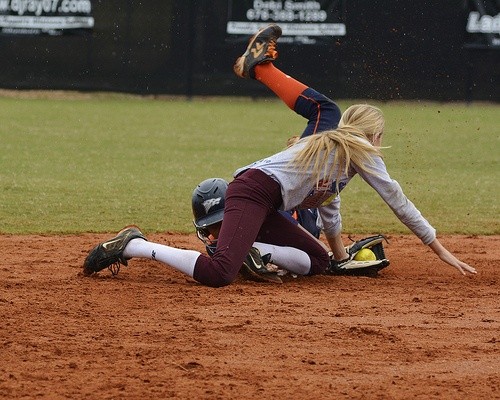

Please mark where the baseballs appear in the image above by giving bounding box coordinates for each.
[354,248,376,262]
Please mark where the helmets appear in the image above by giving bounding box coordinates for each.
[192,178,230,228]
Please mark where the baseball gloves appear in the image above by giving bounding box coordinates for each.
[327,235,390,275]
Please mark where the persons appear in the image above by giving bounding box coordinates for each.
[192,24,340,277]
[83,104,477,289]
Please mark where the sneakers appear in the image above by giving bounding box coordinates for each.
[233,23,283,81]
[207,240,282,284]
[83,224,147,276]
